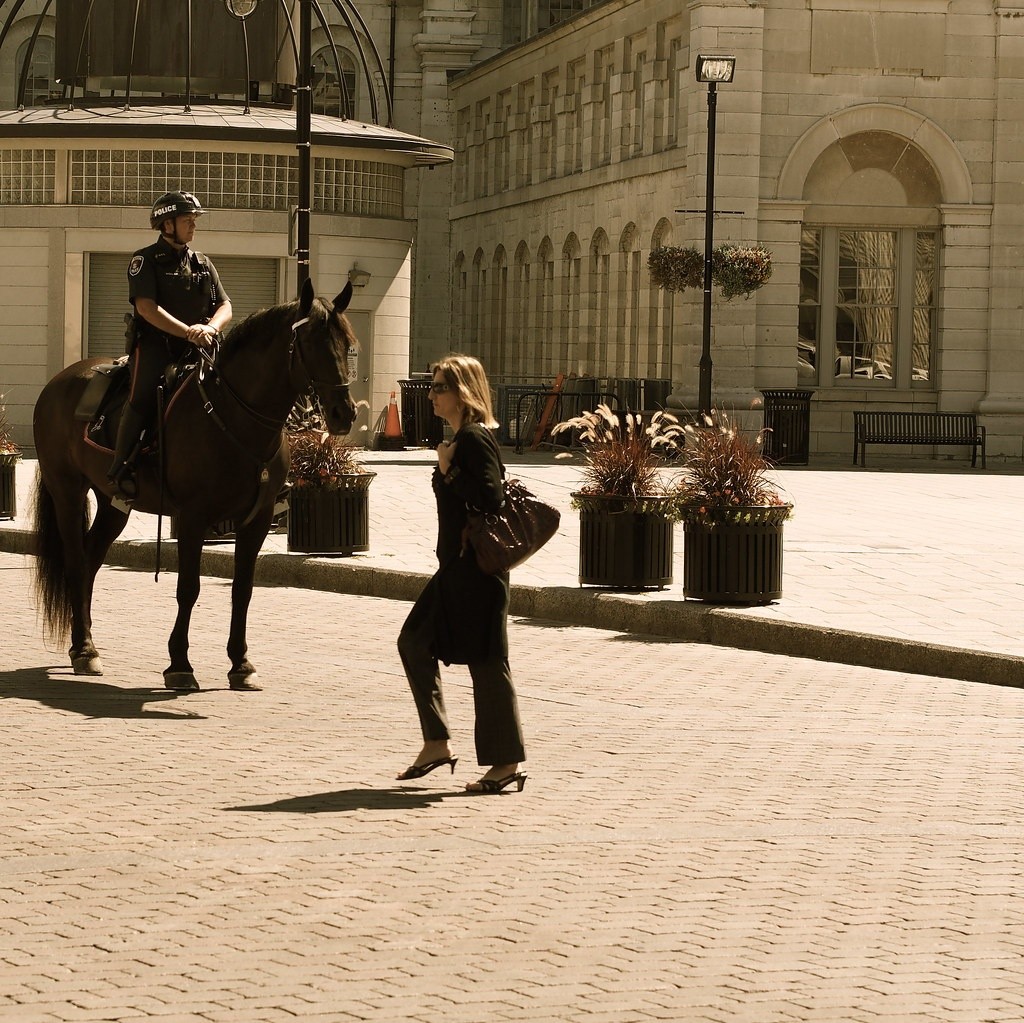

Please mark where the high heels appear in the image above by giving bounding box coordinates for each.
[395,754,458,780]
[466,770,528,791]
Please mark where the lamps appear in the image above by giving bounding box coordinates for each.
[348,269,371,287]
[223,0,259,115]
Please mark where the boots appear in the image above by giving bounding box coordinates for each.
[106,399,147,500]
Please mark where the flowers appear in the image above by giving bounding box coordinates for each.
[711,241,775,303]
[644,239,705,296]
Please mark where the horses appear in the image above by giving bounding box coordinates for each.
[30,277,356,691]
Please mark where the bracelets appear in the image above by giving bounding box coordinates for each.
[207,324,219,337]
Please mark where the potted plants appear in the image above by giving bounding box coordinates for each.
[285,392,377,557]
[551,398,794,604]
[0,394,23,520]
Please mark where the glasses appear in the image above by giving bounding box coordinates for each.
[431,382,452,393]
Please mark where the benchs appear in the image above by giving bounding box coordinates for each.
[852,410,986,472]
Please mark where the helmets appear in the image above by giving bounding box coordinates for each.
[150,191,206,231]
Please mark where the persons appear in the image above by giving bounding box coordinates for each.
[443,439,450,446]
[397,356,528,796]
[106,190,233,486]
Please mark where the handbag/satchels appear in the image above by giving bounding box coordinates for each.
[447,422,561,576]
